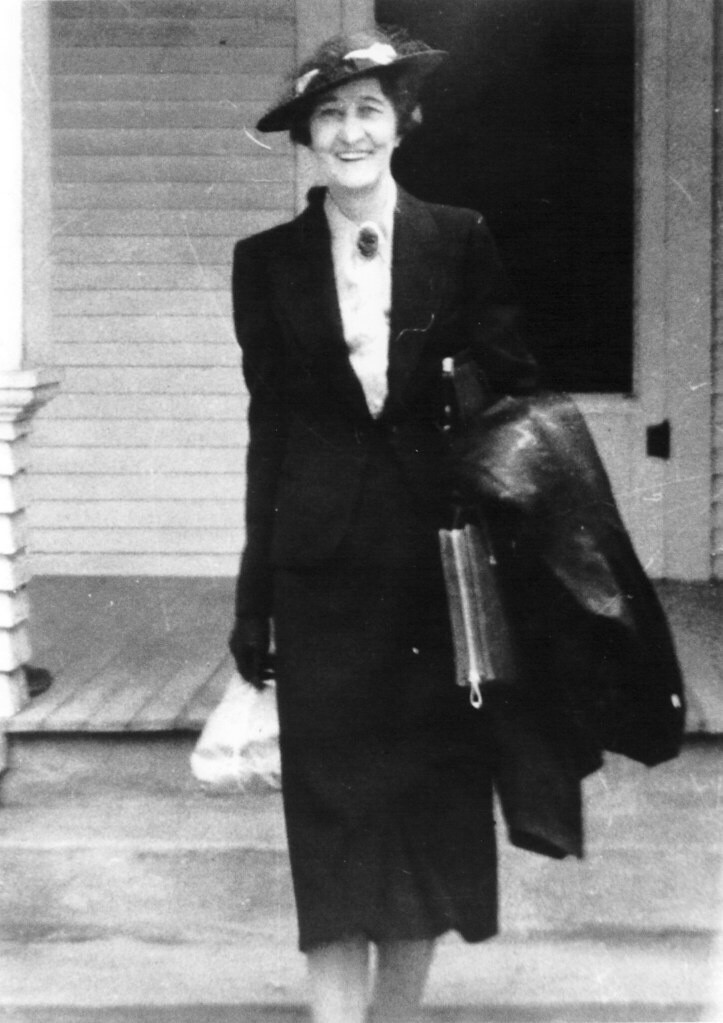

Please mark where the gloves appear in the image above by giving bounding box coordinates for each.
[229,619,276,691]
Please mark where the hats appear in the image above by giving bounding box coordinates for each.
[255,26,452,133]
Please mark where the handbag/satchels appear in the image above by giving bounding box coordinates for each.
[438,520,520,710]
[190,668,281,793]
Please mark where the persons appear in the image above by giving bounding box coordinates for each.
[229,30,566,1023]
